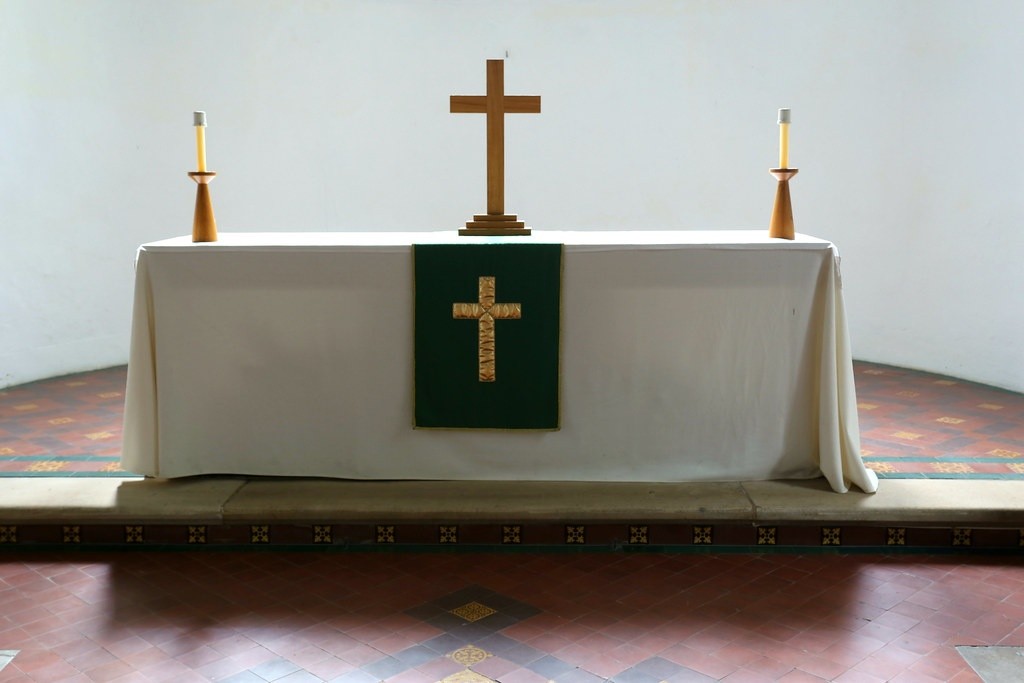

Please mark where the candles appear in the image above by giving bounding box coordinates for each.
[193,110,209,173]
[779,108,792,169]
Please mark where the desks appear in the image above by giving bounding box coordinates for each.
[120,228,882,496]
